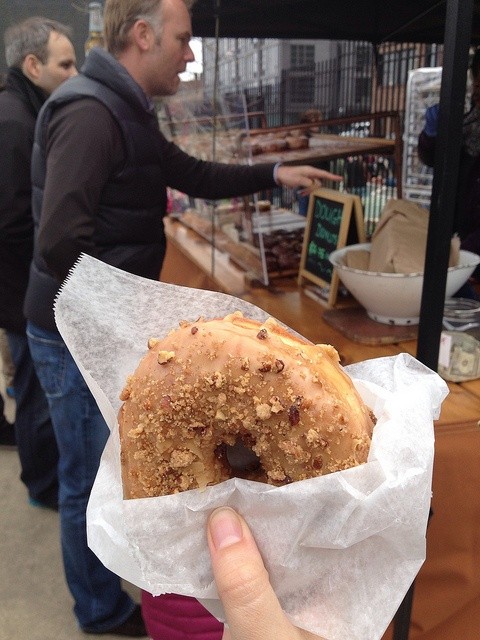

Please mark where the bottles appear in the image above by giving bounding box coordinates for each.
[437,298,480,383]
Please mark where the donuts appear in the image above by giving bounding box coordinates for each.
[117,311,377,499]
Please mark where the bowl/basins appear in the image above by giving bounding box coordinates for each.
[329,242,480,326]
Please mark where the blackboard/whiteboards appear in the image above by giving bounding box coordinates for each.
[300,188,353,292]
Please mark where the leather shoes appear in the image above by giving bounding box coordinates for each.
[28,482,59,511]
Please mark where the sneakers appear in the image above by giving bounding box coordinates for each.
[113,604,146,637]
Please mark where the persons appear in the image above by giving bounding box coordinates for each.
[206,505,348,639]
[19,0,344,639]
[417,48,479,303]
[0,18,82,511]
